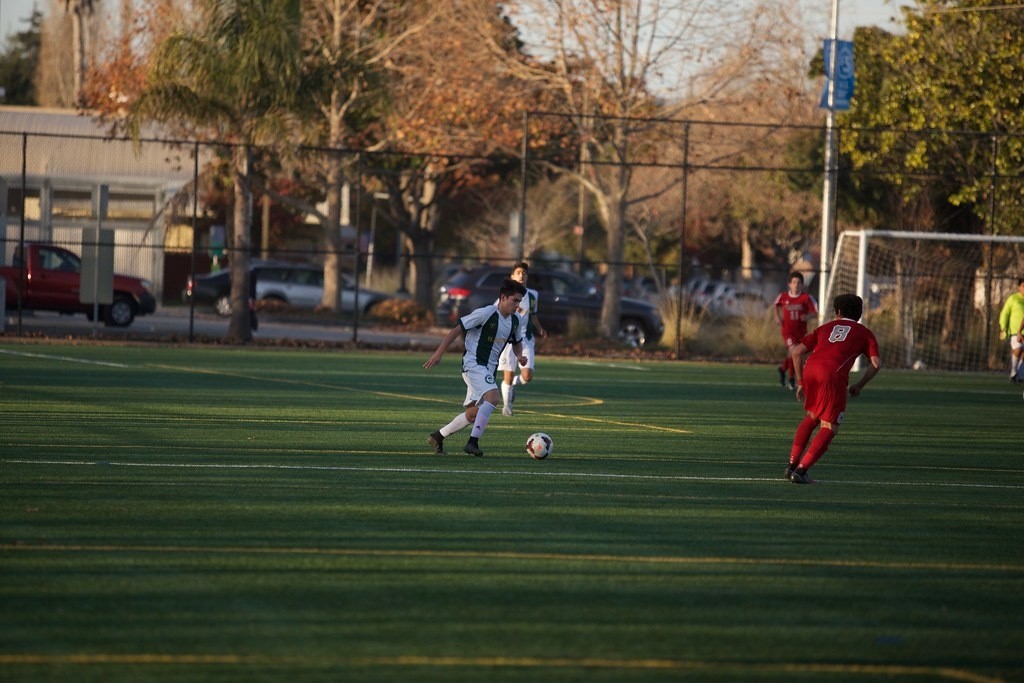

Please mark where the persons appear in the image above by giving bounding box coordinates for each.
[783,293,882,485]
[998,277,1024,385]
[773,272,819,393]
[422,278,528,458]
[491,262,548,417]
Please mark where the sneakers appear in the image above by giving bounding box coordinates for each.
[790,472,819,484]
[463,443,483,456]
[785,463,797,479]
[427,432,448,455]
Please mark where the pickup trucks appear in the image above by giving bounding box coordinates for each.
[2,244,158,331]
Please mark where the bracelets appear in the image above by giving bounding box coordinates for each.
[798,380,804,386]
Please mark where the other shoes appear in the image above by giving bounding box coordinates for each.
[777,367,786,387]
[789,383,795,391]
[502,407,514,416]
[511,386,515,403]
[1010,372,1018,382]
[1017,379,1024,383]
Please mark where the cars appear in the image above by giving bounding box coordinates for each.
[583,269,778,323]
[249,262,407,325]
[181,257,264,319]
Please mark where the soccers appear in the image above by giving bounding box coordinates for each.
[525,432,554,460]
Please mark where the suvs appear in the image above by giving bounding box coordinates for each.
[432,267,667,354]
